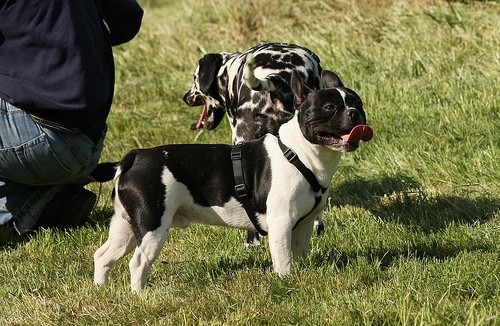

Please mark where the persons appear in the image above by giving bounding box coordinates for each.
[0,0,144,246]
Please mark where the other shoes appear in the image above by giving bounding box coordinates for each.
[40,187,97,229]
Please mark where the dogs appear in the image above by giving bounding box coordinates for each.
[92,69,374,294]
[183,43,325,247]
